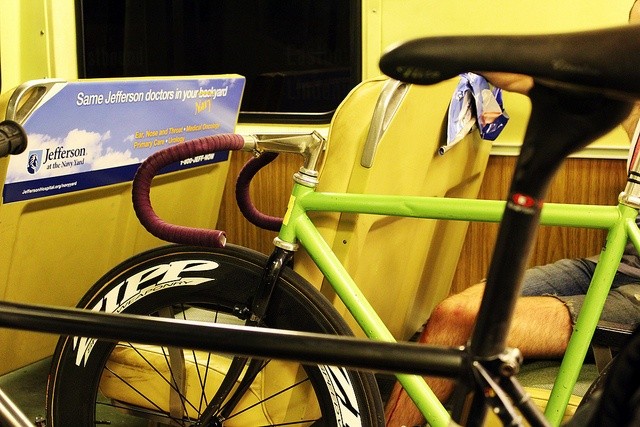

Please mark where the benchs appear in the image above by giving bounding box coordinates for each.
[0,73,247,427]
[484,317,640,427]
[96,73,497,427]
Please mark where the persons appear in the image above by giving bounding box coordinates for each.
[380,0,640,427]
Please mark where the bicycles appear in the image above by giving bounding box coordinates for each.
[0,24,640,427]
[44,130,639,426]
